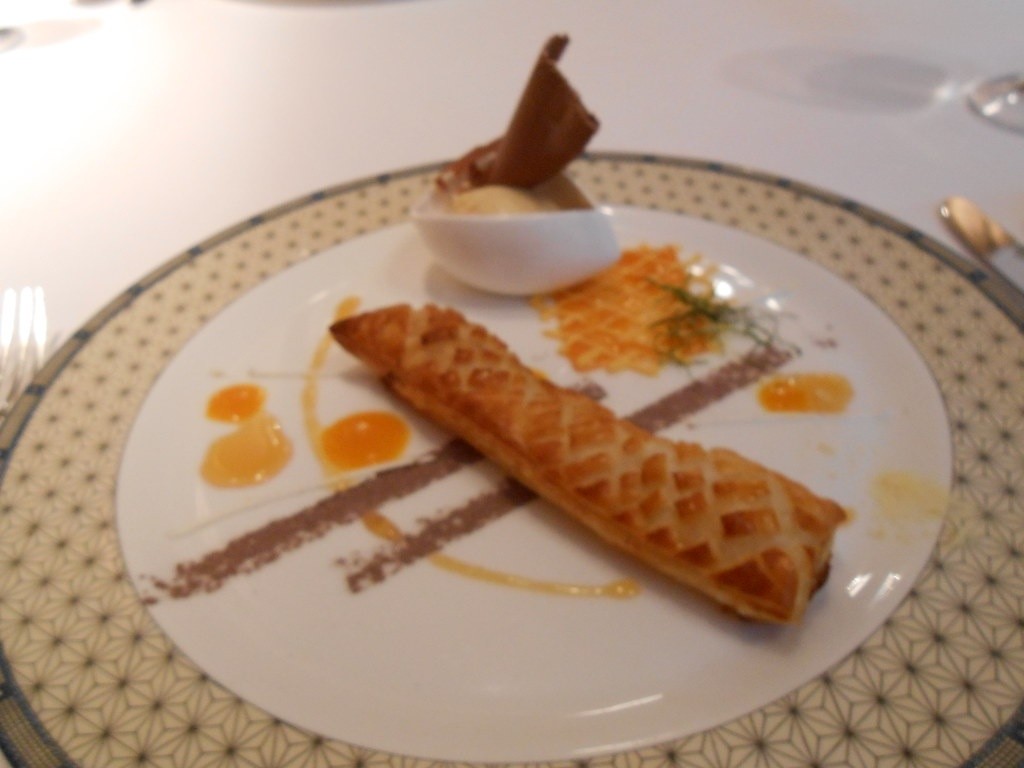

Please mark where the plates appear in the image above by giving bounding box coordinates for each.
[117,205,954,762]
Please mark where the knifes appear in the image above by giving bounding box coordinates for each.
[940,196,1024,290]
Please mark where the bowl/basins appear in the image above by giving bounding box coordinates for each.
[412,177,618,295]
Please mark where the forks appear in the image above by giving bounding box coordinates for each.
[0,283,50,417]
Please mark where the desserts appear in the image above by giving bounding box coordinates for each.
[325,305,848,627]
[433,31,607,220]
